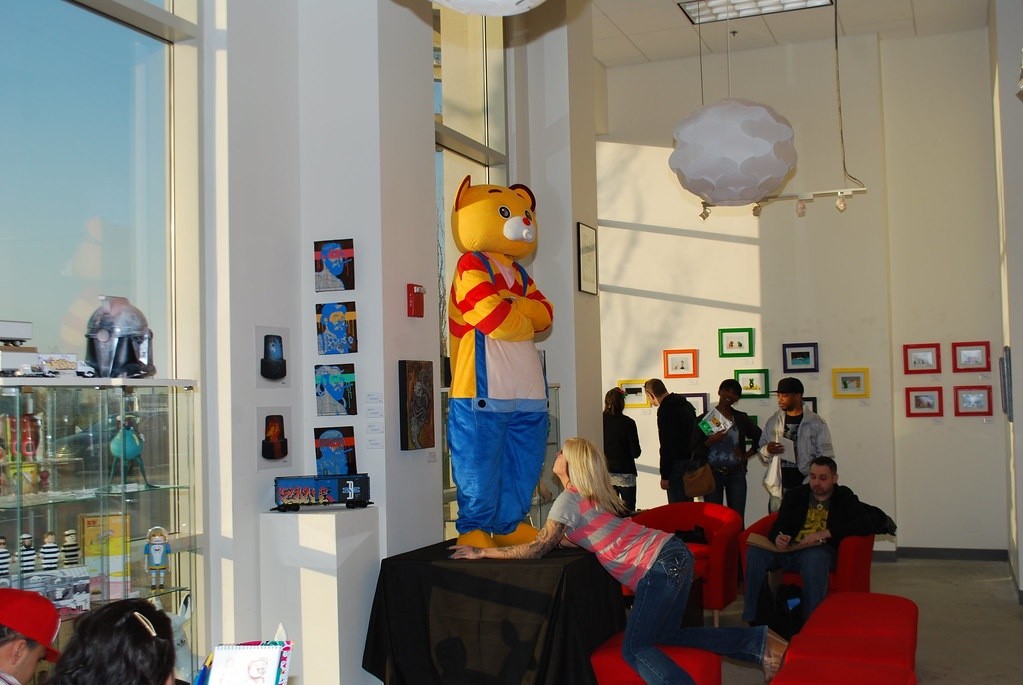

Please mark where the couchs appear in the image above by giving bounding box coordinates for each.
[739,512,875,600]
[622,502,742,627]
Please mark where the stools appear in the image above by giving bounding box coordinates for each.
[768,590,920,685]
[588,629,724,685]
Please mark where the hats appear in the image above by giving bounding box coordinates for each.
[0,587,63,666]
[770,377,805,393]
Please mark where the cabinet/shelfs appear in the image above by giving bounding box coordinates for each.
[0,375,199,685]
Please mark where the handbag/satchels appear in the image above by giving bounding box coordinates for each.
[682,462,716,497]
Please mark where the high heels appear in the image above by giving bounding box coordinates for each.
[762,627,790,683]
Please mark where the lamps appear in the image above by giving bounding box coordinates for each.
[696,0,867,220]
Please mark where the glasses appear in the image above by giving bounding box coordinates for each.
[644,381,654,400]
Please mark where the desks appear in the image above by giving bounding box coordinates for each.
[361,538,627,685]
[260,507,380,683]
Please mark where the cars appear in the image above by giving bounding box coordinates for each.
[45,412,169,475]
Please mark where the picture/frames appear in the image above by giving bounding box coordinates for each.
[951,340,991,372]
[718,328,753,357]
[903,343,941,373]
[679,393,707,416]
[745,416,758,444]
[782,343,818,373]
[905,386,943,417]
[802,396,817,414]
[577,222,598,296]
[663,349,697,378]
[833,367,869,398]
[618,379,650,408]
[953,386,993,417]
[734,369,769,397]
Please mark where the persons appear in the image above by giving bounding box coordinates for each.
[61,528,82,567]
[15,534,37,581]
[753,377,836,515]
[603,385,642,513]
[143,526,172,593]
[643,378,700,506]
[0,588,63,684]
[697,377,763,596]
[39,531,60,571]
[0,536,13,579]
[447,436,792,685]
[48,597,177,685]
[744,455,874,630]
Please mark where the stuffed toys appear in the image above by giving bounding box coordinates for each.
[446,173,555,551]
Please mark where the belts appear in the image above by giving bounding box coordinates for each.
[713,463,744,476]
